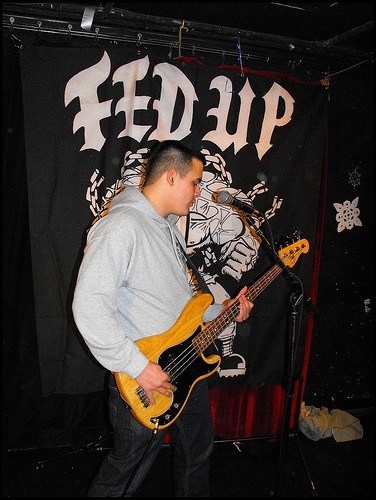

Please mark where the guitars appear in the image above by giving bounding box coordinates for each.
[113,231,310,431]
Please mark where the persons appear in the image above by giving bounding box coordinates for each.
[73,140,255,500]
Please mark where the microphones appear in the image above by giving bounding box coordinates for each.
[216,190,263,217]
